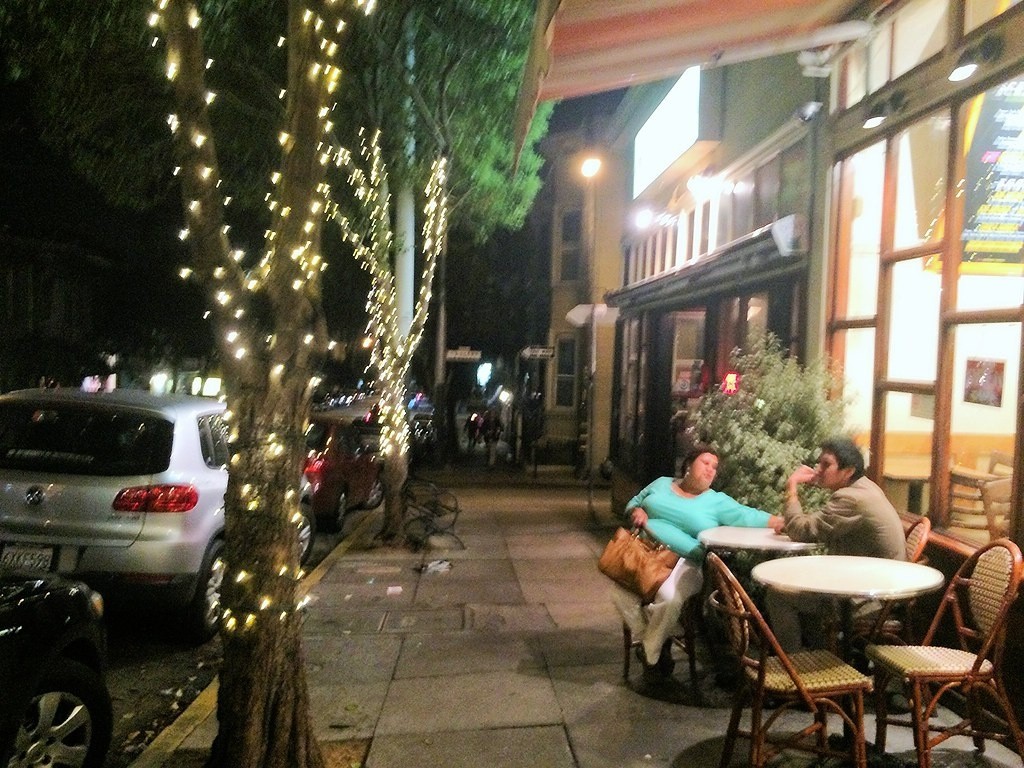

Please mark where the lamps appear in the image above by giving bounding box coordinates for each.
[947,35,1004,82]
[862,90,909,129]
[686,162,720,194]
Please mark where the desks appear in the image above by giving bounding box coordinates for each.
[875,461,932,518]
[698,525,824,656]
[751,555,945,710]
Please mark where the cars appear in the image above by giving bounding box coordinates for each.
[301,390,433,534]
[0,563,119,768]
[0,388,317,642]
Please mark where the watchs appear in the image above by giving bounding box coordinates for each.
[782,489,797,500]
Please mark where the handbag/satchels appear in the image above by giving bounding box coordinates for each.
[595,521,679,598]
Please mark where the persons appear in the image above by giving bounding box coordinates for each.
[762,434,908,653]
[609,446,784,687]
[460,408,503,459]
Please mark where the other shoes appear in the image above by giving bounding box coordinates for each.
[632,645,676,697]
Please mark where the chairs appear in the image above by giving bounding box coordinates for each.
[625,454,1024,768]
